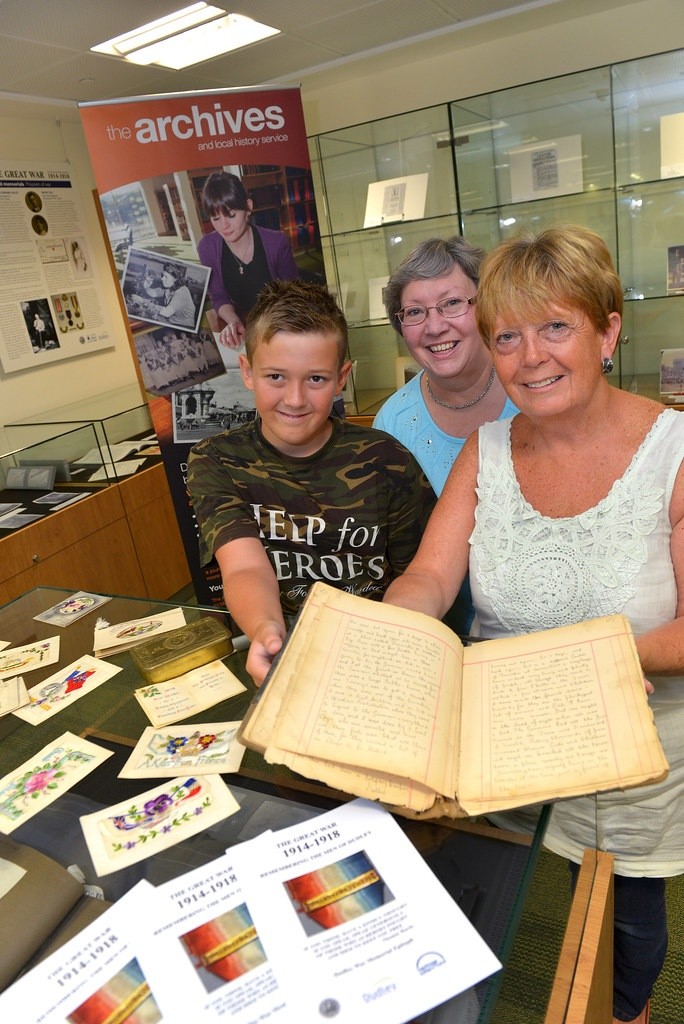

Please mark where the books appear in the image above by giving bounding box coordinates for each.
[235,581,670,821]
[0,797,505,1024]
[74,441,159,463]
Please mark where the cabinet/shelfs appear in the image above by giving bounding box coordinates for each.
[0,47,684,609]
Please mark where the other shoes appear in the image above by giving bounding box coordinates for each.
[645,997,650,1023]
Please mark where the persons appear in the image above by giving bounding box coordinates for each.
[187,277,439,688]
[139,333,213,389]
[33,314,47,348]
[144,261,196,327]
[381,221,684,695]
[371,236,521,501]
[196,172,301,350]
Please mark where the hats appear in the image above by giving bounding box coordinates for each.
[162,262,184,279]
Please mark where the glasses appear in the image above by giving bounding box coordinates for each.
[393,292,477,326]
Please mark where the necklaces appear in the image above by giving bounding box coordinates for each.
[225,226,252,275]
[426,364,495,410]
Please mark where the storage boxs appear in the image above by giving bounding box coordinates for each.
[130,616,234,684]
